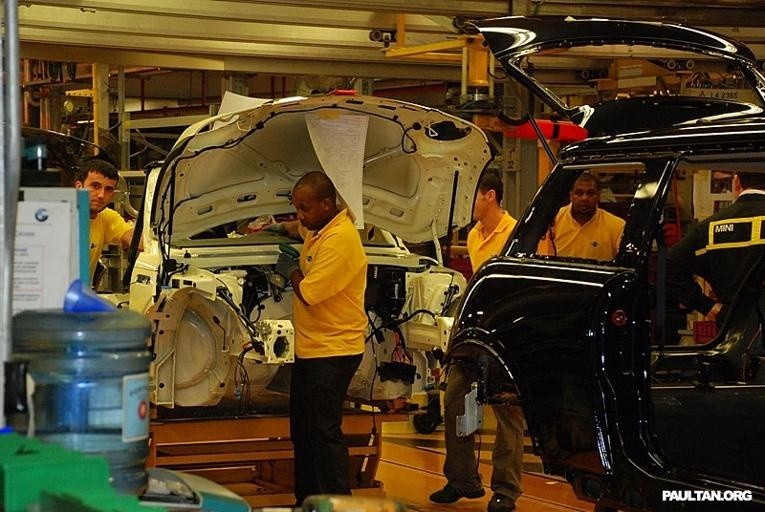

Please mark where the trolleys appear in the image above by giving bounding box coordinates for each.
[407,351,446,435]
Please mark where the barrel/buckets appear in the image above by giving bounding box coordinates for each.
[10,309,156,504]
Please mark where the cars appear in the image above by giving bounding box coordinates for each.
[20,92,495,410]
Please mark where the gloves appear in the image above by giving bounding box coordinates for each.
[276,244,300,280]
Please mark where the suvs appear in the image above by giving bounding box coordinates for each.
[446,16,764,512]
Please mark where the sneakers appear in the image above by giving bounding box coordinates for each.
[428,481,487,506]
[487,492,516,511]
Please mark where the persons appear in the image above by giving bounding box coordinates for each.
[262,171,368,511]
[535,173,627,266]
[70,158,145,292]
[665,170,765,337]
[429,170,526,512]
